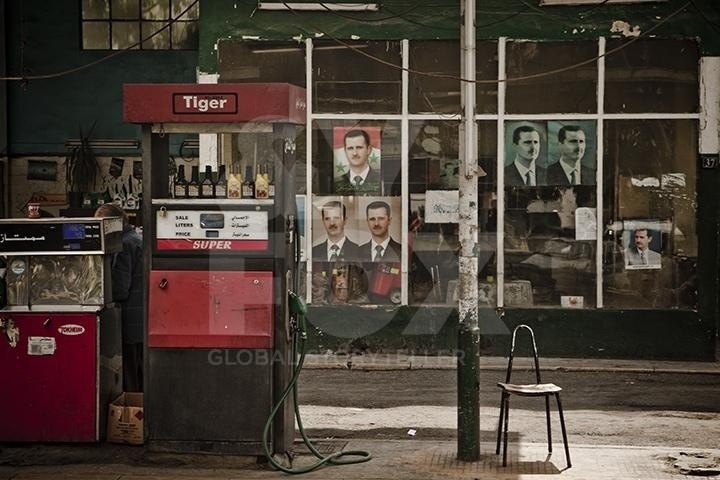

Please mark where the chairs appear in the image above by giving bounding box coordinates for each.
[491,323,571,468]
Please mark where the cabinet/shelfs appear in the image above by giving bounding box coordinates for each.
[1,253,118,441]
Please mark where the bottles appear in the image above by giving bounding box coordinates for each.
[217,163,227,199]
[318,271,330,305]
[330,269,342,304]
[189,165,202,198]
[172,164,188,198]
[485,263,495,282]
[226,162,276,200]
[200,165,216,198]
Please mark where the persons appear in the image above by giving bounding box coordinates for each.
[93,203,144,389]
[359,200,435,289]
[312,201,372,302]
[335,128,382,196]
[410,123,458,177]
[486,199,496,233]
[626,228,661,267]
[502,125,549,189]
[550,125,597,186]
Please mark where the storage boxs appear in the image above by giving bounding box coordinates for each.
[106,388,144,445]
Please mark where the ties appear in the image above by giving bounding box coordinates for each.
[641,252,647,265]
[329,243,340,271]
[525,171,532,185]
[373,245,383,270]
[353,175,363,191]
[570,171,577,185]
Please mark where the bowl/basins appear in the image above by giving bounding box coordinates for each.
[558,295,586,308]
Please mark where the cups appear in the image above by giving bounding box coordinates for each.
[28,202,41,219]
